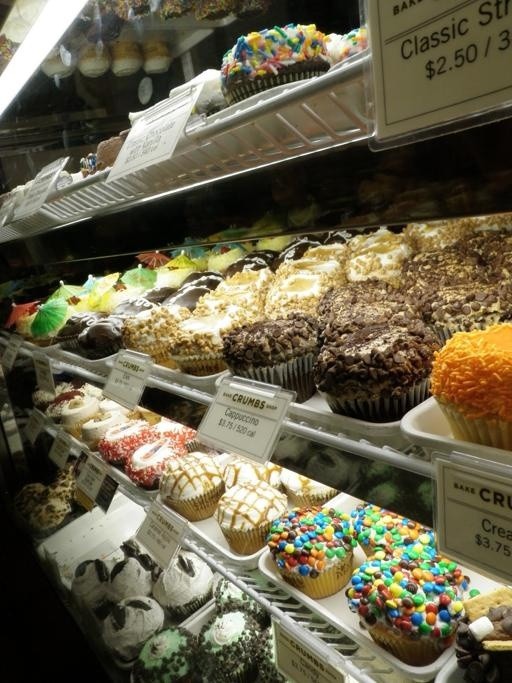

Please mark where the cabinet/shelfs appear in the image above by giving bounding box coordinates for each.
[0,54,512,683]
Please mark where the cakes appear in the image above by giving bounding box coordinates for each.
[214,23,365,108]
[190,176,487,242]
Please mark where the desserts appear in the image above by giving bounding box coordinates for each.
[6,212,511,450]
[25,367,511,681]
